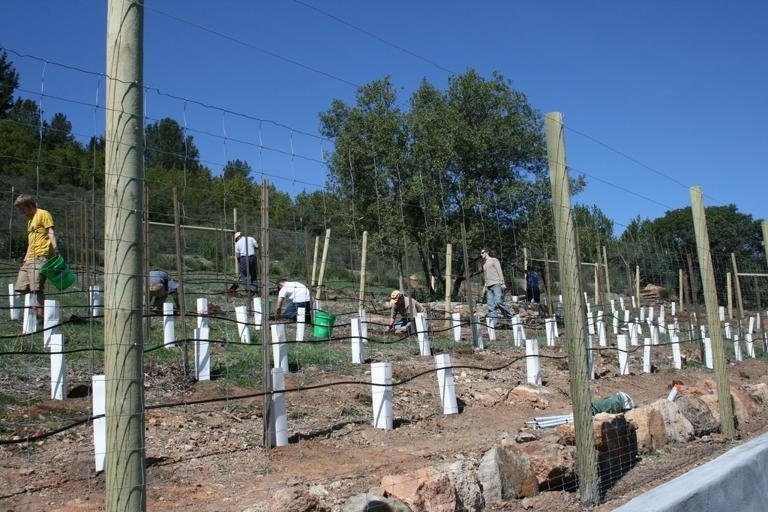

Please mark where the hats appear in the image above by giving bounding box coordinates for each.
[234,232,240,242]
[390,291,403,304]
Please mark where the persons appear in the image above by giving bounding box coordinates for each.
[513,264,540,303]
[384,290,428,336]
[14,193,59,325]
[235,232,259,299]
[481,247,513,328]
[276,279,310,322]
[142,268,180,315]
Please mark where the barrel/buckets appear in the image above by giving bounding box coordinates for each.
[39,254,76,291]
[313,311,335,339]
